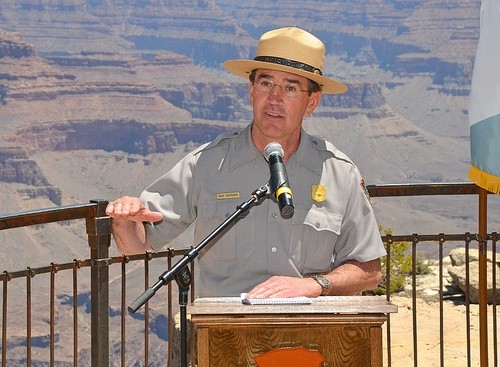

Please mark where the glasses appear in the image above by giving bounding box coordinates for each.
[254,77,309,95]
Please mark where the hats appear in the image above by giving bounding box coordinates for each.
[224,27,348,94]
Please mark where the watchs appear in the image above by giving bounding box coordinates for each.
[312,275,331,296]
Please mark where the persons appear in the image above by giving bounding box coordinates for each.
[105,27,388,299]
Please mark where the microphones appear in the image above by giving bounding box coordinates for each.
[264,142,295,219]
